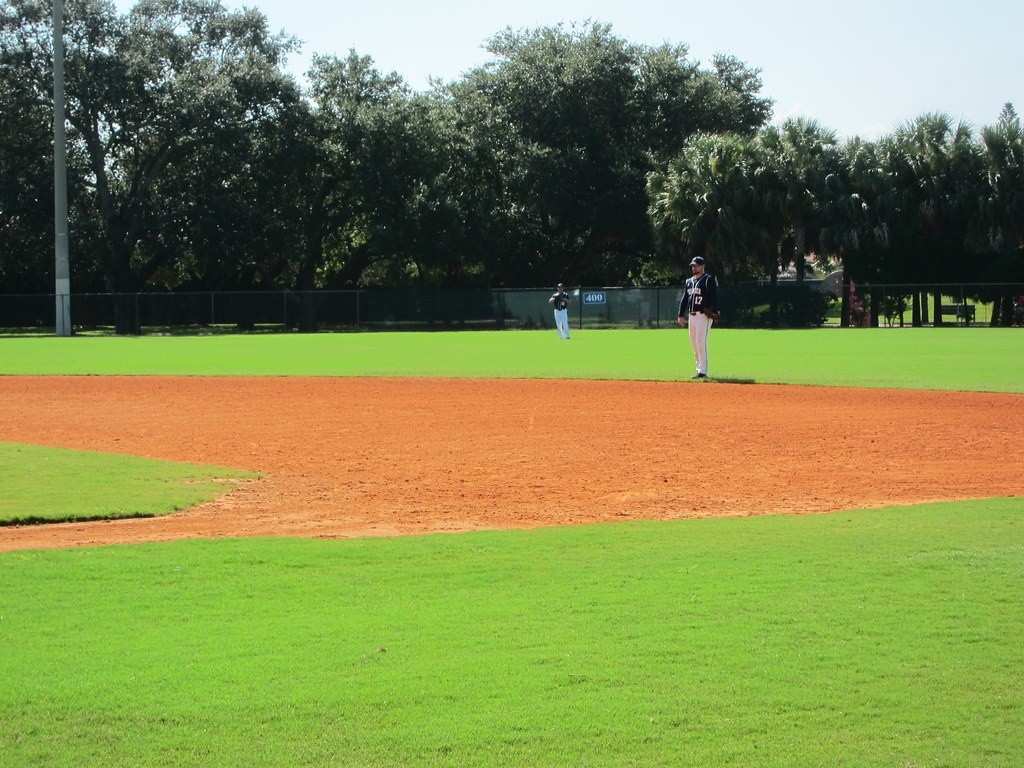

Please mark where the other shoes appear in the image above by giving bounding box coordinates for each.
[566,337,570,339]
[691,374,707,379]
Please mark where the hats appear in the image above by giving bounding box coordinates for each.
[689,256,704,266]
[557,283,564,287]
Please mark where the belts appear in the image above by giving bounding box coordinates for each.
[690,311,705,315]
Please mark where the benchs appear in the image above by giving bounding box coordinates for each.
[941,305,976,323]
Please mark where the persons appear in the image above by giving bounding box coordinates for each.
[677,257,721,380]
[549,283,570,339]
